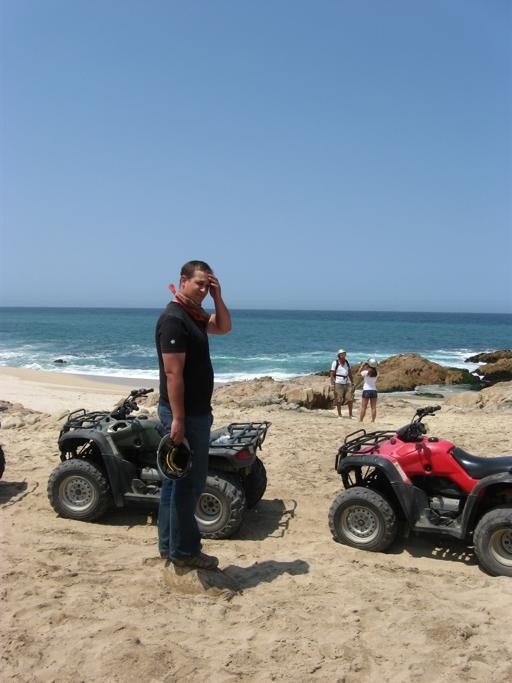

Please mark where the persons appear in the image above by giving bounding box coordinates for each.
[330,349,380,422]
[155,259,231,569]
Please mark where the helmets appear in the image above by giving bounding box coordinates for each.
[337,349,347,356]
[368,358,378,368]
[156,433,193,481]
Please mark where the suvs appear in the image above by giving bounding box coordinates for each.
[47,387,272,539]
[328,405,512,577]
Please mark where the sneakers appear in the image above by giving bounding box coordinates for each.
[159,550,219,569]
